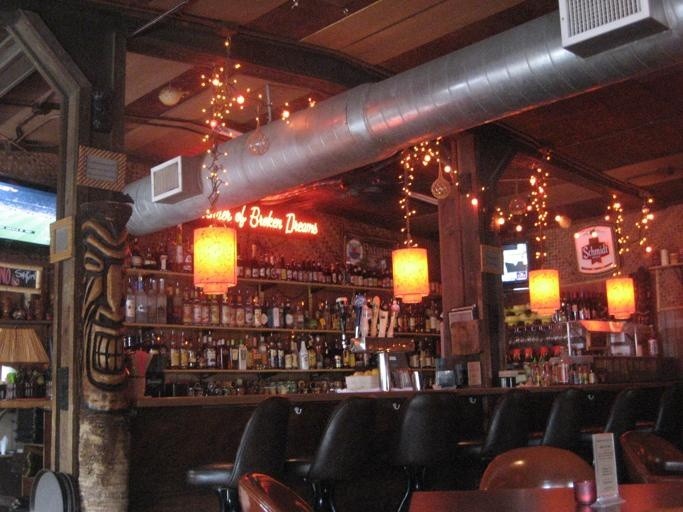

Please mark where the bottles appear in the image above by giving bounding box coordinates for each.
[504,291,660,388]
[122,236,442,397]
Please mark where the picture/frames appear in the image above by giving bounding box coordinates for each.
[0,262,44,295]
[75,145,126,192]
[49,215,75,265]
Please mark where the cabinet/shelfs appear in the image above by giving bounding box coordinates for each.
[0,396,52,497]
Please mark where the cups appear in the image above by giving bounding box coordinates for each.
[574,478,596,504]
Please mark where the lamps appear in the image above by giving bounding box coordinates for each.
[604,190,656,321]
[493,146,562,316]
[389,136,463,306]
[190,34,316,295]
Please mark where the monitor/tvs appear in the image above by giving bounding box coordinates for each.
[502,239,530,285]
[0,175,56,258]
